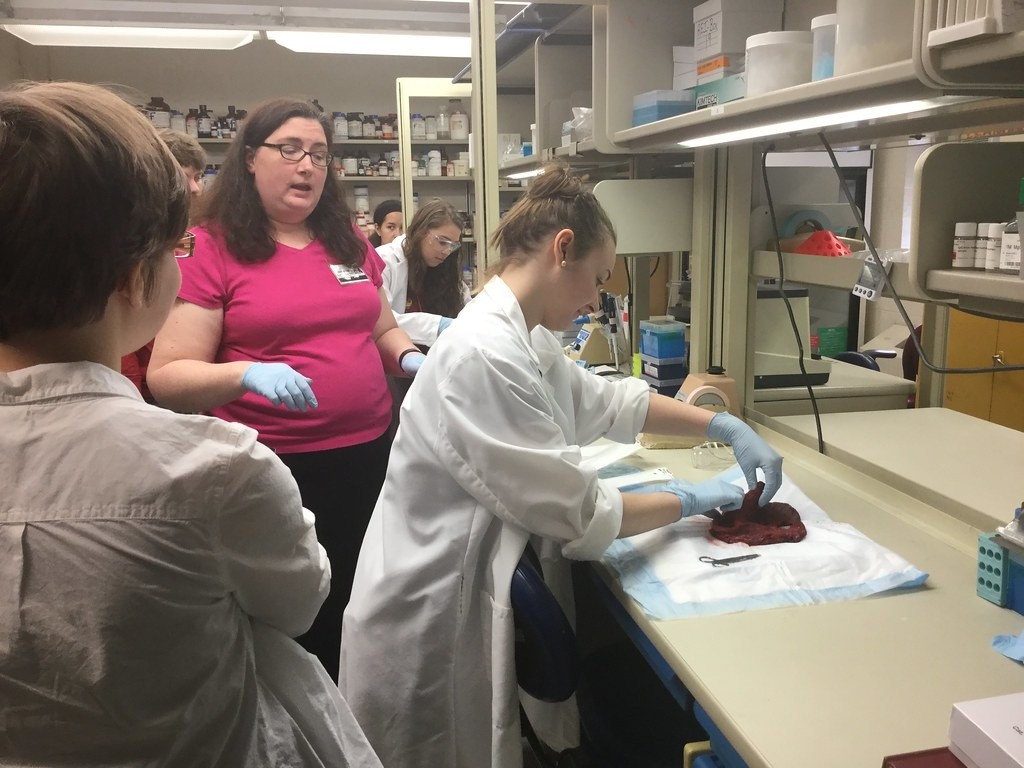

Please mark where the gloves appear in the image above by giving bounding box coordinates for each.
[241,361,318,412]
[661,480,745,522]
[707,410,783,506]
[400,352,426,376]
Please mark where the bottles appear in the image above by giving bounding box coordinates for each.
[744,31,814,97]
[462,266,473,290]
[811,13,837,81]
[530,124,536,155]
[332,147,454,177]
[352,185,376,239]
[952,222,1009,273]
[312,99,469,140]
[202,164,222,193]
[456,210,472,237]
[413,192,419,218]
[135,97,247,139]
[999,177,1024,275]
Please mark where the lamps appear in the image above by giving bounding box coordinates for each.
[0,23,472,58]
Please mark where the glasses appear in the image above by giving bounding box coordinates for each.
[168,231,196,258]
[258,143,335,167]
[428,230,461,252]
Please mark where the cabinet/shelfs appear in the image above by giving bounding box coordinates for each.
[195,136,476,244]
[449,0,1024,768]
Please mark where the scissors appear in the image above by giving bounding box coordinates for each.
[699,555,761,568]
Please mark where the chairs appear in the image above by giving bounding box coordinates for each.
[512,541,613,768]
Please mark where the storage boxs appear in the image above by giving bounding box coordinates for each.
[686,72,745,108]
[697,55,746,86]
[640,373,685,398]
[633,90,696,127]
[692,0,785,62]
[639,353,684,381]
[640,321,686,358]
[948,692,1024,768]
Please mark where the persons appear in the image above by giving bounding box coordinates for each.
[141,99,426,691]
[370,195,472,444]
[0,76,388,768]
[121,129,208,399]
[367,199,405,248]
[336,162,785,768]
